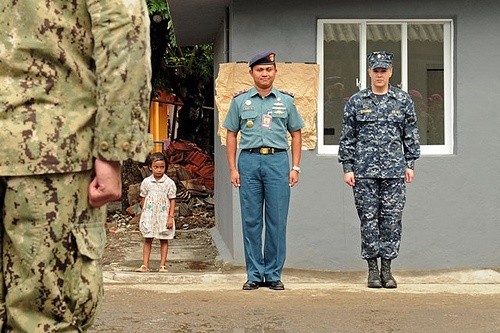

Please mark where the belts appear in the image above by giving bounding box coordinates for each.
[244,146,286,155]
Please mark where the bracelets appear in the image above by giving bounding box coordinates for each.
[292,166,302,173]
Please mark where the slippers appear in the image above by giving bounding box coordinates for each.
[158,265,167,272]
[136,266,149,272]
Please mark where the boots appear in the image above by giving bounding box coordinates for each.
[366,258,382,288]
[380,257,397,287]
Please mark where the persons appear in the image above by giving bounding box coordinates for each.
[0,0,152,333]
[222,51,305,290]
[324,83,445,145]
[339,51,420,288]
[134,152,177,273]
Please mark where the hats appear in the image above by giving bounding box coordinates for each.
[248,52,275,66]
[367,51,393,69]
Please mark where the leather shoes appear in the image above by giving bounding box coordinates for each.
[265,279,284,290]
[244,280,258,290]
